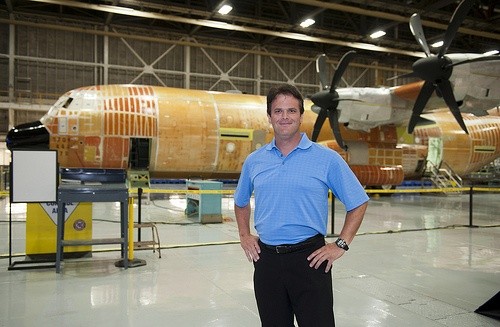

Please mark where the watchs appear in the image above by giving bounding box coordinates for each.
[335,238,349,251]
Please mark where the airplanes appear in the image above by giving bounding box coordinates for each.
[9,0,500,196]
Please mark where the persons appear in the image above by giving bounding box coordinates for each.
[233,84,370,327]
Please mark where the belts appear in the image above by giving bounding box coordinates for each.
[258,234,324,254]
